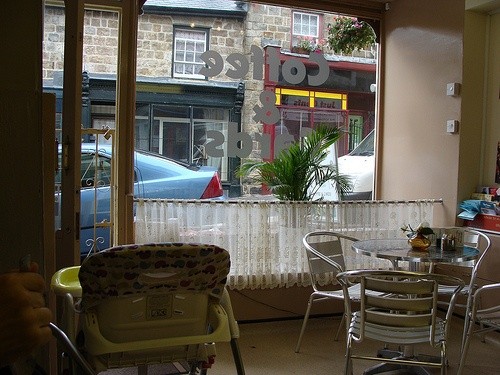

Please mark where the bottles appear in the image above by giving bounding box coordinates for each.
[407,232,431,251]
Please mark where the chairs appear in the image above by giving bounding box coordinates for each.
[296,228,500,375]
[50,241,246,375]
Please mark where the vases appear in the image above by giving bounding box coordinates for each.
[292,48,321,54]
[409,232,432,249]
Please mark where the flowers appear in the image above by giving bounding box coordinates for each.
[297,35,325,50]
[401,222,434,236]
[326,16,375,56]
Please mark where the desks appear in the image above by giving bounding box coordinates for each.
[351,238,481,375]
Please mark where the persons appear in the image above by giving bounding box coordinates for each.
[0,261,53,371]
